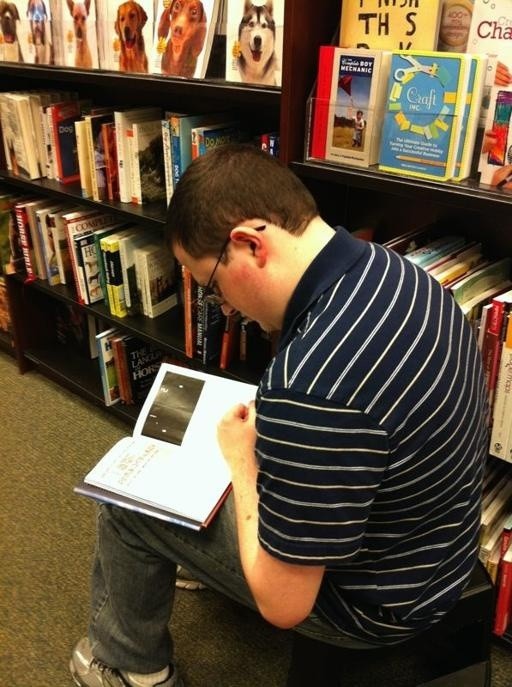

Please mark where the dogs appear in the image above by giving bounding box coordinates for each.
[235,0,282,87]
[26,0,54,64]
[158,0,207,79]
[66,0,92,70]
[0,0,24,64]
[114,0,148,74]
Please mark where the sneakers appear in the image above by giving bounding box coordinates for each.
[68,637,185,687]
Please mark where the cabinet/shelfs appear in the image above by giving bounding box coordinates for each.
[0,1,282,430]
[281,0,511,651]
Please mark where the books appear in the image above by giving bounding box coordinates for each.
[69,305,171,406]
[0,90,239,207]
[74,362,259,532]
[0,189,285,365]
[311,0,512,187]
[0,0,284,87]
[349,222,512,464]
[477,464,512,635]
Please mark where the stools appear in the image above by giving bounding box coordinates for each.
[286,559,496,687]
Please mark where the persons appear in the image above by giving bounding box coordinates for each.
[71,142,487,687]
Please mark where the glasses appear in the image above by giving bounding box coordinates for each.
[200,224,266,306]
